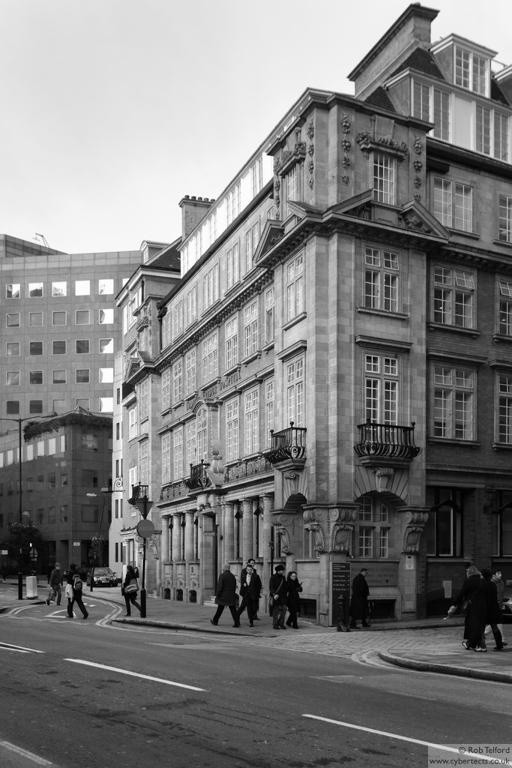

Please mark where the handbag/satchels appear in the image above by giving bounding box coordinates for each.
[124,578,139,593]
[269,597,275,610]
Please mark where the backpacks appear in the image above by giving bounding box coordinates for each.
[69,571,82,590]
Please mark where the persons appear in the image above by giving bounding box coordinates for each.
[348,569,371,629]
[209,564,240,627]
[285,570,303,629]
[479,570,504,650]
[238,557,258,620]
[242,565,262,626]
[484,569,510,646]
[456,562,489,652]
[46,562,64,605]
[268,564,288,630]
[67,564,88,619]
[122,565,142,616]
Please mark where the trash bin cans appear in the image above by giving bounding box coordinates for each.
[26,576,38,599]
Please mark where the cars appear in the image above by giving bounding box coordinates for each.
[87,567,118,587]
[497,582,512,620]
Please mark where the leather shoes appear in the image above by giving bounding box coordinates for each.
[252,616,259,620]
[210,619,217,626]
[250,624,254,627]
[232,624,239,627]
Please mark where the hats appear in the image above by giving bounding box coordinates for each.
[222,564,230,570]
[275,565,285,570]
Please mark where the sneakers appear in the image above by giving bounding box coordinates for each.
[124,613,130,616]
[462,640,469,649]
[475,647,487,652]
[494,642,507,649]
[272,625,285,629]
[67,615,72,618]
[349,625,360,630]
[286,622,298,628]
[82,613,88,619]
[362,624,371,627]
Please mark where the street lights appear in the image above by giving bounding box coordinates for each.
[135,485,153,617]
[0,412,57,599]
[85,493,106,591]
[30,548,38,576]
